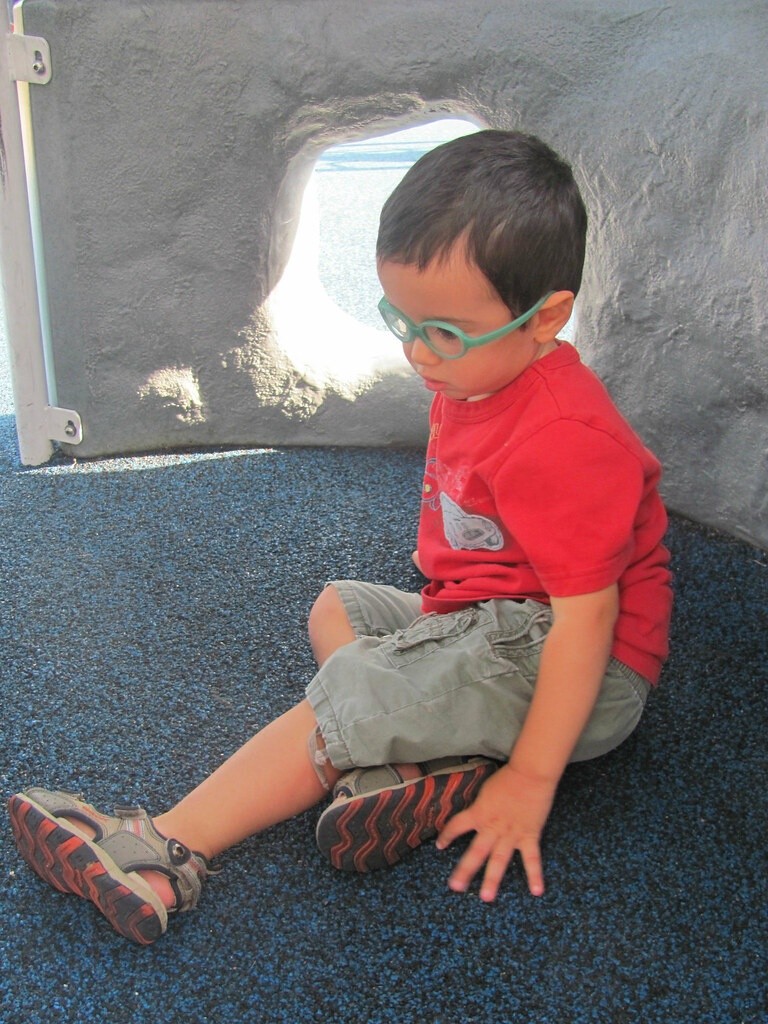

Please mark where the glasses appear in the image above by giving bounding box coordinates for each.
[377,290,556,360]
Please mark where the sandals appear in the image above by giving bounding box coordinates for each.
[9,788,226,945]
[316,754,498,873]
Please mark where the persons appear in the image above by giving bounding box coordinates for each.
[9,129,672,944]
